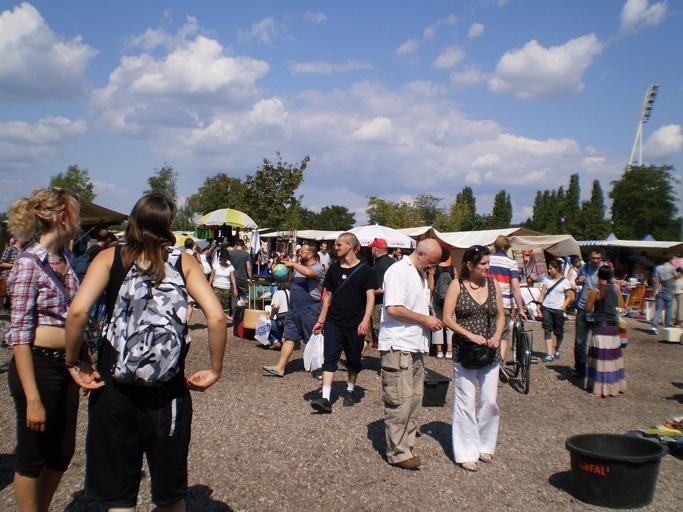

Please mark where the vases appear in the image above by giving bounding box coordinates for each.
[563,433,666,509]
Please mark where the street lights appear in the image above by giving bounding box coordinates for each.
[626,83,659,171]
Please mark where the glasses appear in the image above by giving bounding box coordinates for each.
[469,246,489,259]
[297,249,311,254]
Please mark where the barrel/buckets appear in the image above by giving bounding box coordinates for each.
[565,434,669,508]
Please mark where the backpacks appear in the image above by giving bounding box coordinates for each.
[433,272,452,308]
[96,255,196,392]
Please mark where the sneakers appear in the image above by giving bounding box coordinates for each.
[650,328,659,335]
[269,342,281,349]
[554,347,561,359]
[342,390,355,406]
[310,398,332,413]
[445,351,453,360]
[437,351,444,358]
[262,366,283,377]
[543,355,553,362]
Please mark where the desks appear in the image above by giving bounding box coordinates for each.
[246,277,278,310]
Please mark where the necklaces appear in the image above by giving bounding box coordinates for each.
[56,255,63,265]
[468,277,483,290]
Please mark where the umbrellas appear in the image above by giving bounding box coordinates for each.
[343,222,417,249]
[196,207,258,228]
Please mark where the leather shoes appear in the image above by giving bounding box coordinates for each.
[389,457,421,469]
[478,454,492,464]
[460,463,477,472]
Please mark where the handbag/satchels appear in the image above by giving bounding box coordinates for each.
[617,319,629,349]
[458,342,496,370]
[276,317,286,327]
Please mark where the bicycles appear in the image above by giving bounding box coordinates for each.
[505,300,542,392]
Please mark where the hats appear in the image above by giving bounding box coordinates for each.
[367,239,387,249]
[569,255,579,264]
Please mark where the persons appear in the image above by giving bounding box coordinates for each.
[65,193,227,511]
[376,238,445,468]
[2,188,87,511]
[255,241,336,377]
[1,234,27,318]
[184,235,252,323]
[567,255,585,293]
[427,247,457,359]
[537,260,574,362]
[443,243,506,473]
[490,236,527,361]
[355,239,410,356]
[575,247,626,396]
[632,263,648,278]
[309,233,376,414]
[649,255,682,334]
[77,233,119,282]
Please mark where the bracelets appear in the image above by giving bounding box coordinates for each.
[318,321,324,325]
[66,360,80,369]
